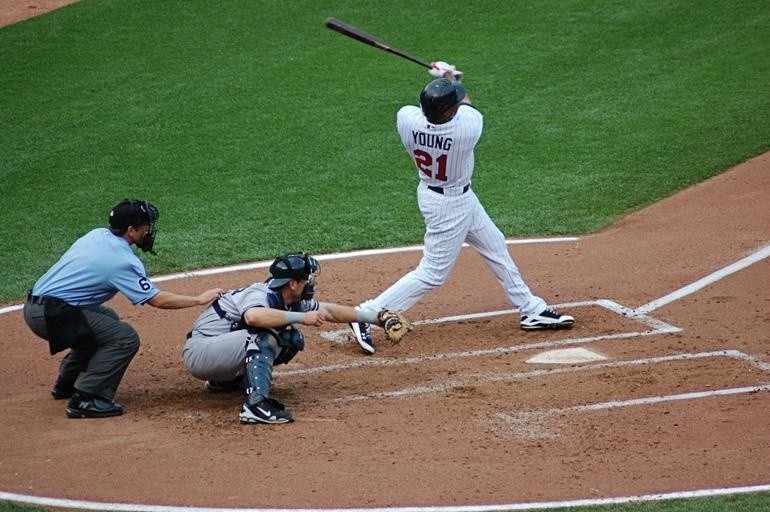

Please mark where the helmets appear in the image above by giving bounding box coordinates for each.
[420,79,465,122]
[107,199,158,257]
[268,252,321,300]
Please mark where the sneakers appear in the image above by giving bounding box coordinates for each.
[519,309,576,331]
[65,393,123,419]
[350,320,375,354]
[50,375,78,397]
[204,380,235,393]
[237,401,294,425]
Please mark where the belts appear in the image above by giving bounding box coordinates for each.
[25,288,43,305]
[428,186,470,196]
[186,331,192,339]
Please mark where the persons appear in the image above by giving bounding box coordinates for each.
[182,249,414,425]
[348,58,581,355]
[20,197,226,418]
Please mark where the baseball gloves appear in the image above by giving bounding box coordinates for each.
[377,307,413,344]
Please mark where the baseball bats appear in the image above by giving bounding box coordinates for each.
[325,18,432,70]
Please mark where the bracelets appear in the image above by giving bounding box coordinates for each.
[286,310,305,324]
[356,308,377,324]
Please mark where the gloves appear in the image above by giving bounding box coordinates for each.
[429,62,464,82]
